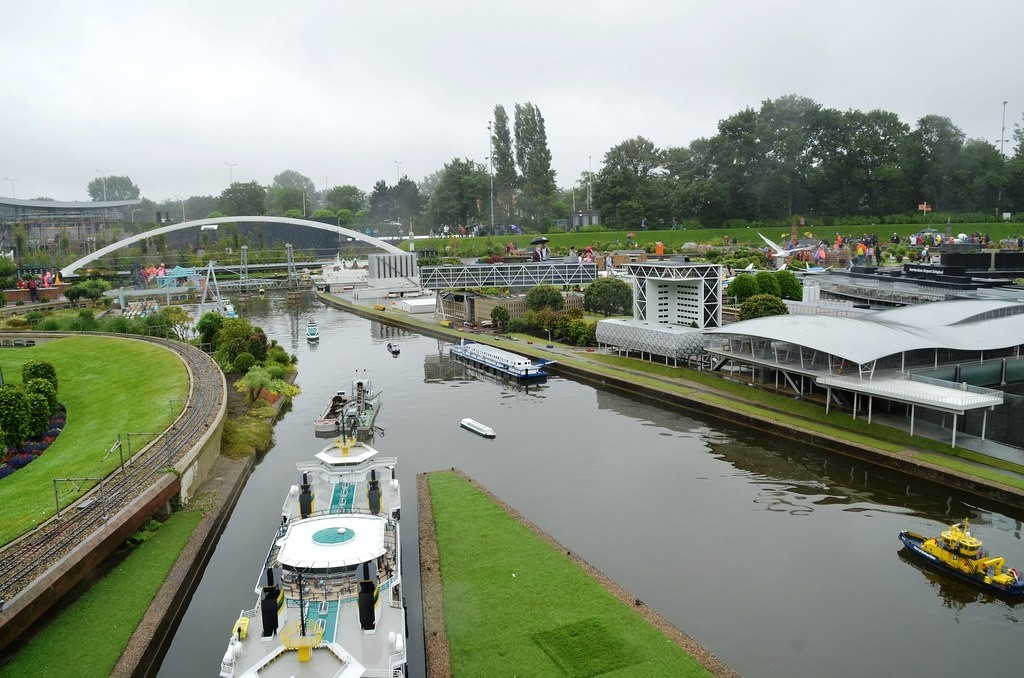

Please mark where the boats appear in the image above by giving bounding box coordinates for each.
[219,409,410,678]
[460,417,496,438]
[306,322,320,340]
[899,516,1024,598]
[315,368,382,434]
[387,343,400,354]
[450,338,556,385]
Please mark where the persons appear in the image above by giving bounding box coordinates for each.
[130,260,166,289]
[891,232,942,263]
[504,241,519,253]
[1016,230,1024,247]
[533,244,550,262]
[659,219,677,231]
[570,246,599,262]
[948,229,989,252]
[429,223,524,238]
[843,233,882,266]
[18,268,64,303]
[768,249,773,261]
[356,226,379,239]
[642,218,648,230]
[615,236,638,250]
[789,233,842,261]
[606,252,614,277]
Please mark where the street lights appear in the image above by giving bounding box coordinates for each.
[174,195,185,222]
[131,208,141,223]
[95,170,111,219]
[225,163,236,185]
[394,160,403,182]
[484,120,494,229]
[545,329,550,343]
[296,188,306,217]
[4,177,21,199]
[995,139,1010,201]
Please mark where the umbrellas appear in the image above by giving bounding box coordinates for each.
[627,233,636,237]
[530,237,549,245]
